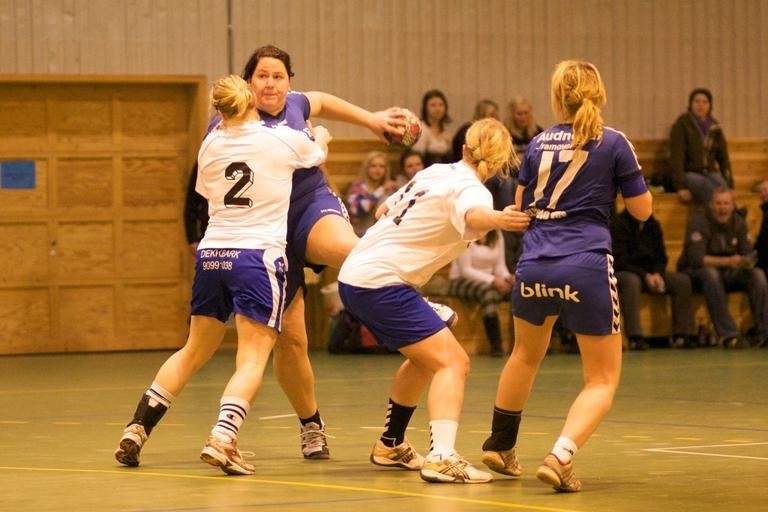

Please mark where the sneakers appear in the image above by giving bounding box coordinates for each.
[420,454,494,484]
[371,439,425,469]
[201,435,256,476]
[115,422,147,465]
[482,448,524,476]
[536,454,581,492]
[423,295,457,328]
[301,421,330,459]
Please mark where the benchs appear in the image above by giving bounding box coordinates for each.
[305,130,766,353]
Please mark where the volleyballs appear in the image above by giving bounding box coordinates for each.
[384,109,423,149]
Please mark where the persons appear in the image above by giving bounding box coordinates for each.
[450,97,545,209]
[346,90,455,218]
[482,62,653,492]
[204,45,458,459]
[611,208,700,352]
[449,229,582,356]
[113,75,333,475]
[664,91,733,204]
[746,202,767,278]
[337,117,530,483]
[677,186,767,350]
